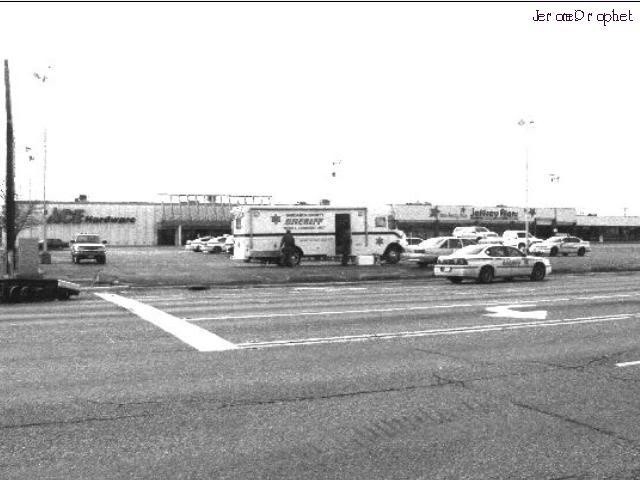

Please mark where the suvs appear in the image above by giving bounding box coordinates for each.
[70,232,108,265]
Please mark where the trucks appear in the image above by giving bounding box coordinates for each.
[230,205,408,268]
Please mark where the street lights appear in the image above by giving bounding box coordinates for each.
[518,118,532,246]
[33,69,52,264]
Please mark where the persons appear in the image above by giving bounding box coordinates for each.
[280,230,295,265]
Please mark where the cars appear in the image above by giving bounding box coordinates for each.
[433,243,552,283]
[406,226,544,266]
[39,238,70,251]
[184,235,234,254]
[528,234,591,257]
